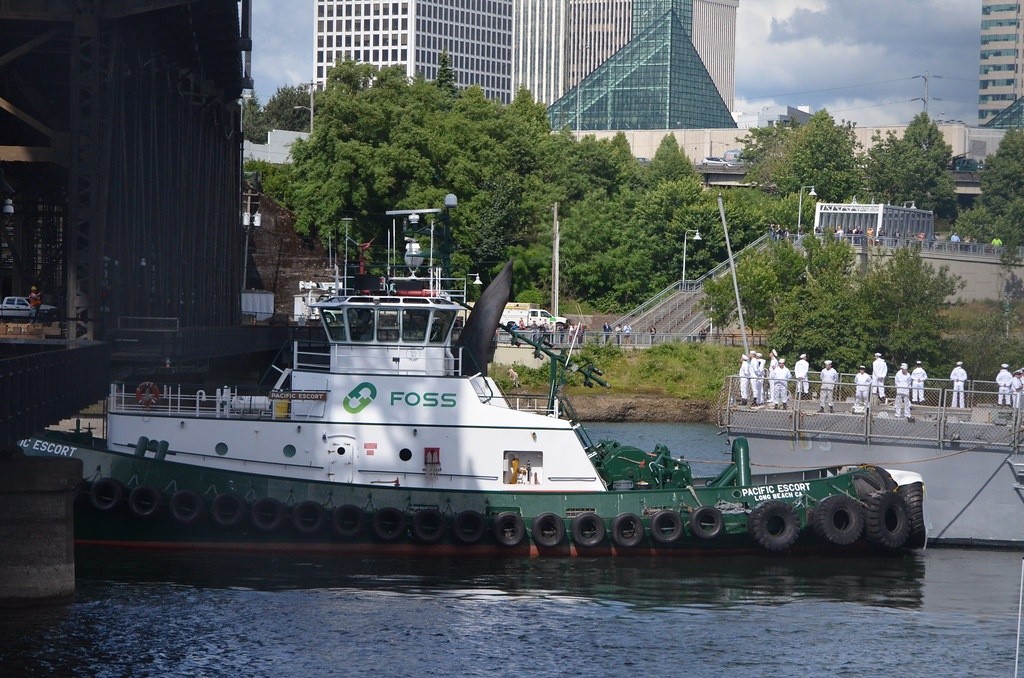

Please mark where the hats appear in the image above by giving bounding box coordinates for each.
[742,354,749,361]
[750,350,755,355]
[916,360,922,365]
[900,363,909,369]
[858,365,866,369]
[799,353,806,358]
[1012,369,1023,374]
[824,360,833,366]
[956,361,963,366]
[778,359,785,364]
[875,353,881,357]
[772,349,778,357]
[1001,363,1010,369]
[756,353,763,357]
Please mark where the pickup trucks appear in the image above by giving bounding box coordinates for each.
[0,297,58,320]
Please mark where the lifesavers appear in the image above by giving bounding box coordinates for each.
[532,513,566,547]
[868,491,910,547]
[897,481,927,549]
[332,505,366,538]
[495,511,524,547]
[211,493,244,526]
[293,501,326,534]
[252,498,284,530]
[169,492,203,521]
[613,513,644,548]
[749,500,801,550]
[129,487,159,516]
[453,511,485,544]
[652,506,723,542]
[413,509,447,542]
[89,478,122,511]
[814,495,864,545]
[573,513,605,546]
[372,508,405,539]
[137,382,159,404]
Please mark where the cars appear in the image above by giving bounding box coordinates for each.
[636,157,652,163]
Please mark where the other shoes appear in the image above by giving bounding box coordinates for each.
[829,406,833,413]
[817,407,824,413]
[772,404,778,409]
[782,403,787,410]
[738,398,747,405]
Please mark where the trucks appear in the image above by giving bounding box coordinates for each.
[458,302,569,331]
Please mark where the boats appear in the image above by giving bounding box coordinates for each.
[16,192,926,563]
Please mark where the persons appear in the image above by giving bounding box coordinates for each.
[739,349,792,410]
[794,353,810,399]
[769,222,1003,254]
[894,360,928,418]
[950,362,967,408]
[854,365,872,413]
[818,360,838,414]
[509,369,519,387]
[649,325,656,346]
[872,353,888,404]
[996,364,1024,410]
[603,322,632,345]
[28,286,42,323]
[698,325,707,342]
[510,318,587,344]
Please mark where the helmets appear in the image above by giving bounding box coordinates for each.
[31,286,37,290]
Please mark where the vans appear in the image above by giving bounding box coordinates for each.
[724,150,747,166]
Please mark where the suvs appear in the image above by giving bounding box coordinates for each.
[702,158,731,167]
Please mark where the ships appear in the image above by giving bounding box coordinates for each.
[716,368,1024,547]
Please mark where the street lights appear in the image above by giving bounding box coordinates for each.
[464,273,483,328]
[341,217,354,296]
[293,106,313,135]
[236,88,254,285]
[797,185,817,238]
[682,228,702,283]
